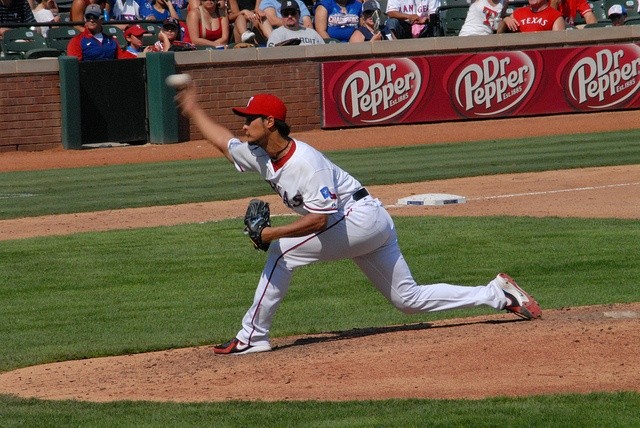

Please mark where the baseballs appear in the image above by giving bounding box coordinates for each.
[164,73,192,87]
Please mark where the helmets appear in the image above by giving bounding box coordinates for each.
[362,0,382,14]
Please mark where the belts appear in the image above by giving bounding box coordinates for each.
[352,188,369,201]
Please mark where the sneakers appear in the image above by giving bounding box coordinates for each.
[214,337,272,356]
[496,271,542,320]
[241,30,256,43]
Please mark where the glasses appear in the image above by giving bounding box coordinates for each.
[283,11,299,16]
[85,14,100,20]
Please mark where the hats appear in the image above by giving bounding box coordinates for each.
[163,16,179,28]
[84,3,103,17]
[608,4,628,19]
[281,0,300,11]
[124,24,149,38]
[232,93,287,121]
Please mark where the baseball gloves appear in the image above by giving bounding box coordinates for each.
[243,199,271,252]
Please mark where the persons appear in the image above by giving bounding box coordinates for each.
[183,1,230,43]
[606,4,628,26]
[459,0,509,37]
[547,0,599,25]
[266,0,324,45]
[174,78,543,353]
[145,0,178,21]
[153,17,194,52]
[67,4,122,61]
[385,0,445,39]
[350,0,399,42]
[497,0,563,33]
[32,0,61,39]
[0,0,41,45]
[231,0,274,42]
[122,24,153,60]
[259,0,312,29]
[312,0,362,43]
[72,0,110,32]
